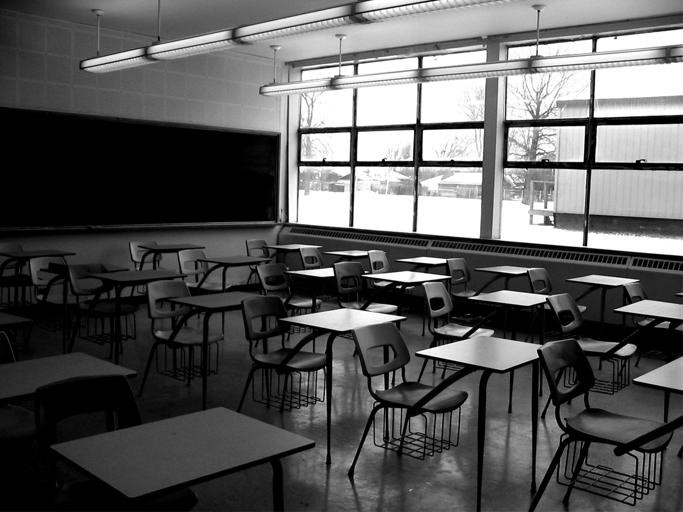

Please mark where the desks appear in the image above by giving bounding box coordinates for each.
[632,357,683,395]
[48,402,317,512]
[564,272,640,368]
[467,289,549,413]
[414,335,547,512]
[277,306,408,465]
[614,297,683,421]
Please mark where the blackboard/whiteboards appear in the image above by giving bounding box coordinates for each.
[0,105,281,233]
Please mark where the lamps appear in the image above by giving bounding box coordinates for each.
[80,0,518,75]
[260,2,683,96]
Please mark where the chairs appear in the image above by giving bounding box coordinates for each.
[625,283,683,368]
[526,265,588,332]
[32,372,201,507]
[235,296,328,411]
[529,337,675,511]
[540,293,638,418]
[245,239,531,349]
[415,282,495,383]
[348,322,468,475]
[1,239,342,446]
